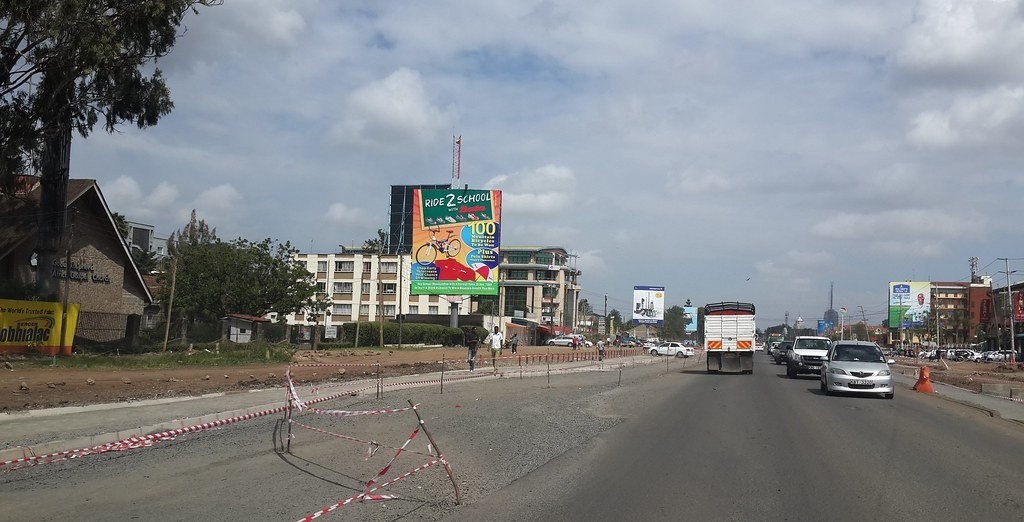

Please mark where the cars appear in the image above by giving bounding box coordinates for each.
[755,343,764,352]
[648,342,694,358]
[819,341,895,399]
[786,336,833,379]
[775,341,794,365]
[621,337,654,350]
[919,349,1017,363]
[545,336,574,348]
[882,348,895,356]
[770,341,780,357]
[576,339,593,348]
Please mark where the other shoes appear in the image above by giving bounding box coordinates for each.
[494,369,498,375]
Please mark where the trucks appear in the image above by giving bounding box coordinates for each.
[766,334,785,354]
[704,301,758,375]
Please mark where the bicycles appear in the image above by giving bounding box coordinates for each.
[415,226,461,266]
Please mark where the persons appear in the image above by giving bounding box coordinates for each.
[950,349,975,360]
[510,333,519,354]
[998,351,1007,362]
[577,336,581,350]
[466,327,481,373]
[616,339,620,349]
[597,338,603,361]
[904,294,930,323]
[573,336,576,350]
[897,347,913,356]
[487,325,503,374]
[918,348,948,362]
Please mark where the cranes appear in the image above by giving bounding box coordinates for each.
[451,135,462,189]
